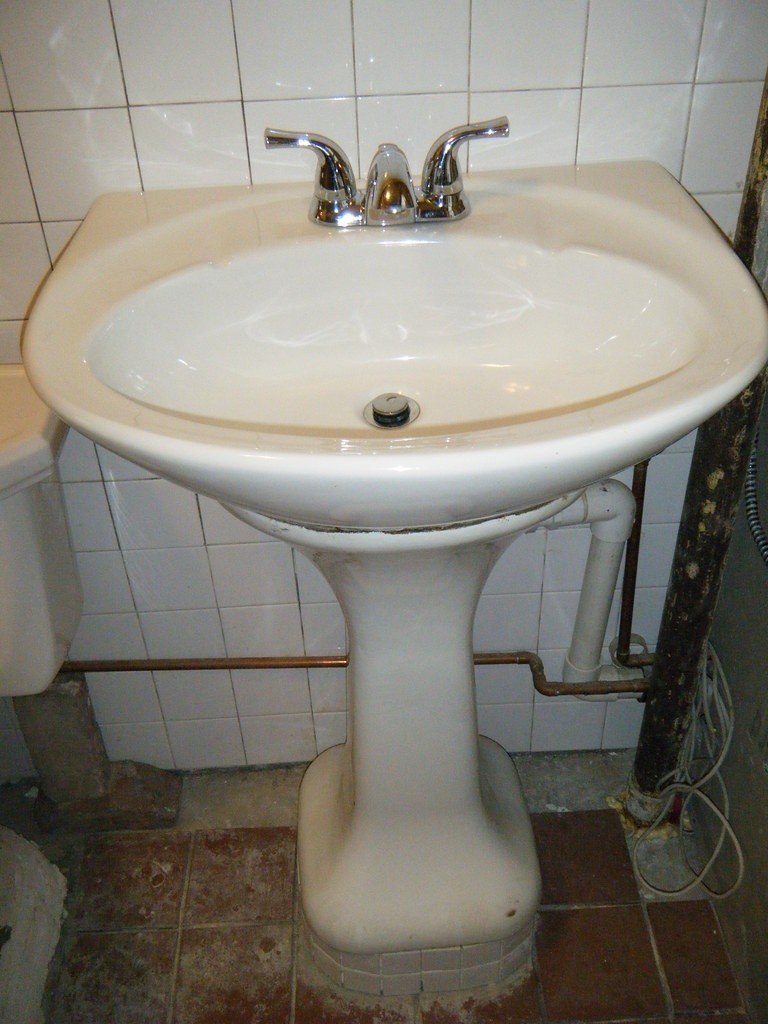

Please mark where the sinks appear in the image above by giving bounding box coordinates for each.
[18,158,767,536]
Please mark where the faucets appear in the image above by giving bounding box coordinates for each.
[359,139,419,225]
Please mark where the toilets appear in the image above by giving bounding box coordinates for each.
[0,358,79,699]
